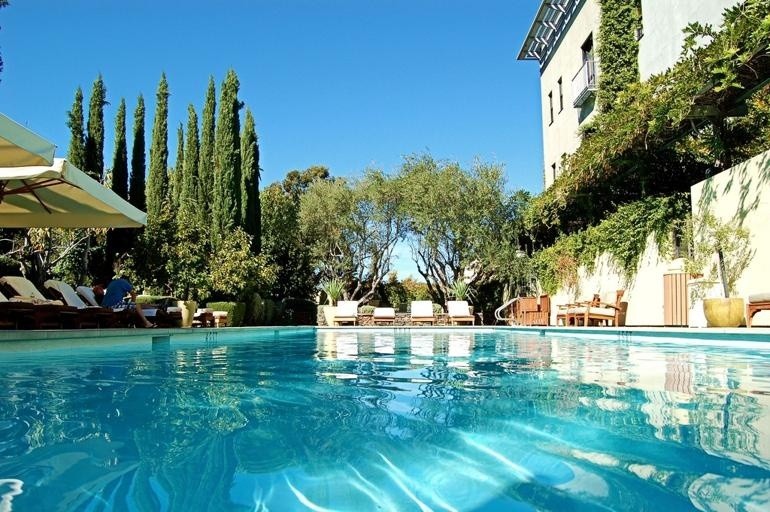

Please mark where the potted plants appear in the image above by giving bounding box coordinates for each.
[676,212,756,327]
[165,245,208,328]
[322,279,347,326]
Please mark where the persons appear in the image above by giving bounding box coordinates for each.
[101,275,159,328]
[93,287,105,306]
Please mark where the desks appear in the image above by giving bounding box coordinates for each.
[433,313,448,326]
[357,312,373,326]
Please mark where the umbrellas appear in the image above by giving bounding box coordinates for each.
[0,112,148,230]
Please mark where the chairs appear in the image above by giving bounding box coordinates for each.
[746,293,770,328]
[333,301,359,326]
[411,300,435,325]
[374,308,395,324]
[447,301,475,326]
[556,289,624,328]
[0,275,228,329]
